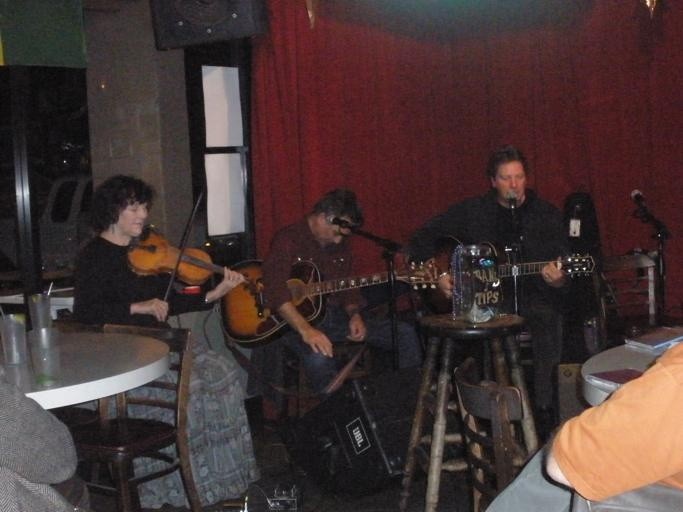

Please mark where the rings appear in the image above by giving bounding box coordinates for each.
[361,334,366,340]
[544,275,551,280]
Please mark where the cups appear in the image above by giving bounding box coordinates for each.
[0,313,29,365]
[28,327,61,387]
[27,294,51,330]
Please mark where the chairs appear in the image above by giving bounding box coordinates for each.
[45,318,205,512]
[593,252,657,322]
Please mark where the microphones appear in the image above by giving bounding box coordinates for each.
[631,188,652,219]
[326,213,349,228]
[505,191,520,220]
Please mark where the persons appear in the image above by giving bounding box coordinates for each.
[543,340,683,511]
[407,143,583,444]
[259,186,426,401]
[70,173,264,512]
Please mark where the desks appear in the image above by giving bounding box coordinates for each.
[580,343,660,409]
[0,329,170,411]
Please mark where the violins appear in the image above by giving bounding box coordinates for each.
[127,228,260,303]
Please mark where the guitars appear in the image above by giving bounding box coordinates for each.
[407,232,596,314]
[222,255,440,347]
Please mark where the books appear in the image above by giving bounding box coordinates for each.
[625,326,683,350]
